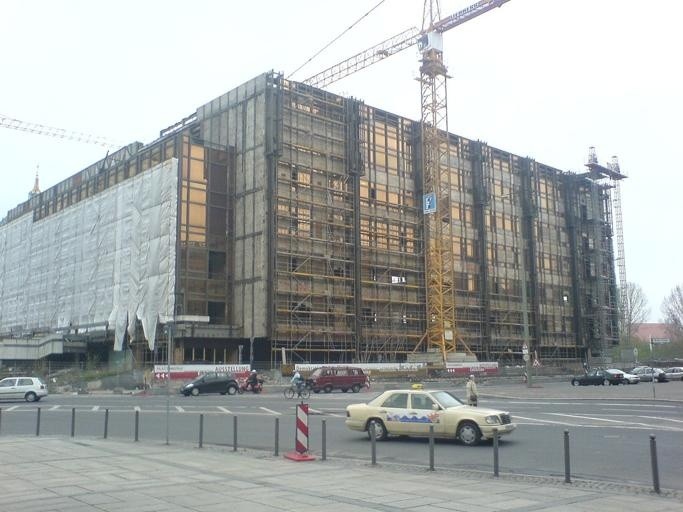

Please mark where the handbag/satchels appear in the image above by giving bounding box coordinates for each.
[470,395,477,401]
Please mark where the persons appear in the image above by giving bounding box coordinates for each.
[465,375,479,407]
[288,369,302,399]
[245,369,257,389]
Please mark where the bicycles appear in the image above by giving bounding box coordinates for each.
[284,382,310,399]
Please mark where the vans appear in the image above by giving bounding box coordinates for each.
[311,367,365,393]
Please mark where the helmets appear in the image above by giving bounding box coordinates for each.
[252,369,257,373]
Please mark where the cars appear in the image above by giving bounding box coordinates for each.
[180,371,239,396]
[345,390,516,445]
[571,367,683,386]
[0,376,48,402]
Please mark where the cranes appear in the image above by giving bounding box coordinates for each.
[606,154,629,345]
[287,0,507,359]
[1,115,116,147]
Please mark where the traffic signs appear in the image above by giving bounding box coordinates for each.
[652,338,672,343]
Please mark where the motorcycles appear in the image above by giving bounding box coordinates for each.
[238,378,262,394]
